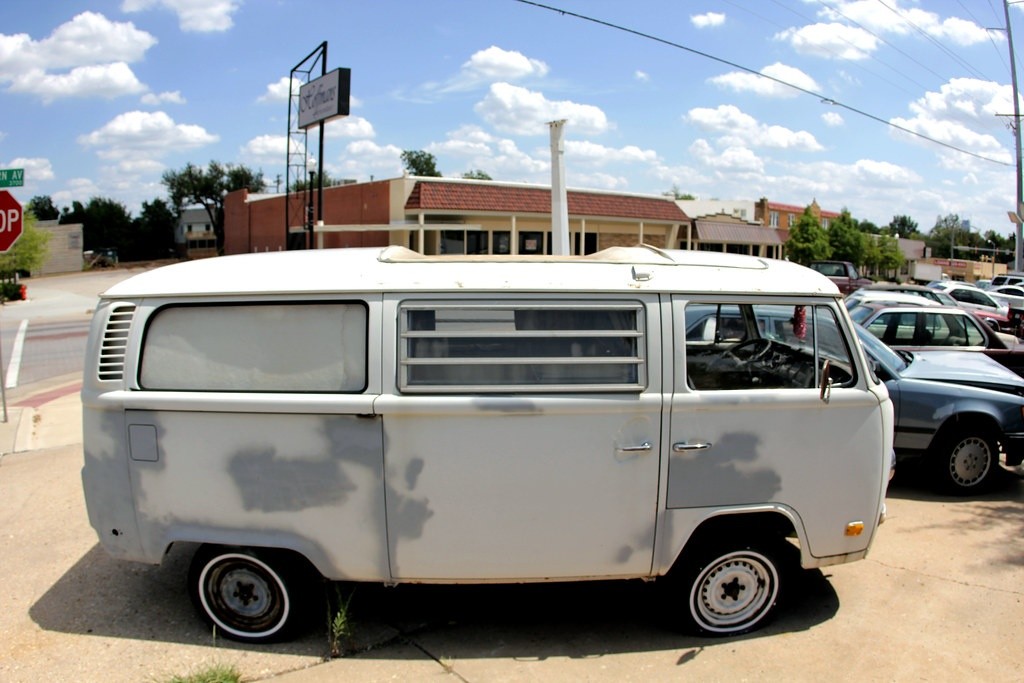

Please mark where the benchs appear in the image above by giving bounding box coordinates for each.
[943,335,978,346]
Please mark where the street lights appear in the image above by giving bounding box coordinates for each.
[987,239,995,279]
[307,150,317,250]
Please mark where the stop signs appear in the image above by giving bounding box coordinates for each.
[0,188,23,253]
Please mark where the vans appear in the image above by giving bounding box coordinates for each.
[79,243,897,647]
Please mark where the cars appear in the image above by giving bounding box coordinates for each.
[847,304,1024,380]
[681,305,1024,499]
[801,258,1024,378]
